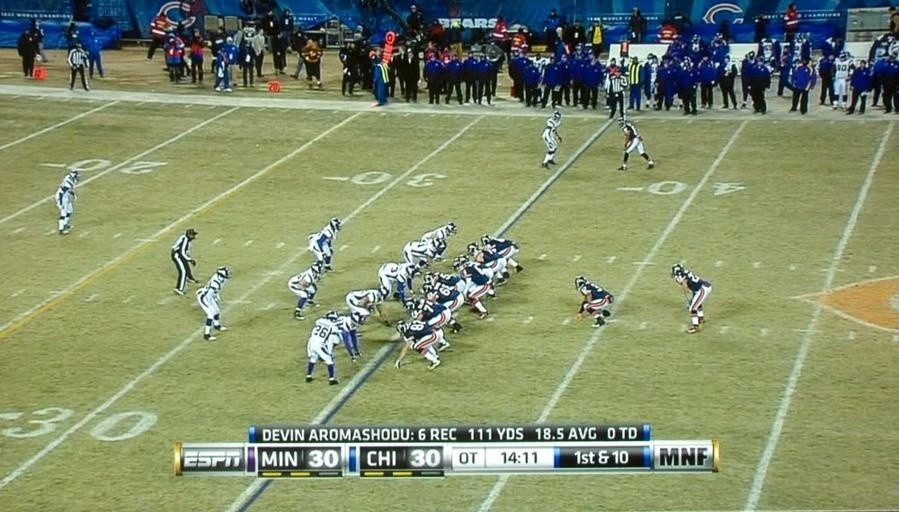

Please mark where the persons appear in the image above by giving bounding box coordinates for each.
[13,3,899,122]
[318,308,369,362]
[344,218,525,370]
[302,308,343,386]
[615,117,656,173]
[53,168,81,235]
[306,216,344,275]
[572,273,616,330]
[166,226,199,297]
[196,263,232,342]
[283,259,330,321]
[668,262,714,335]
[540,108,563,170]
